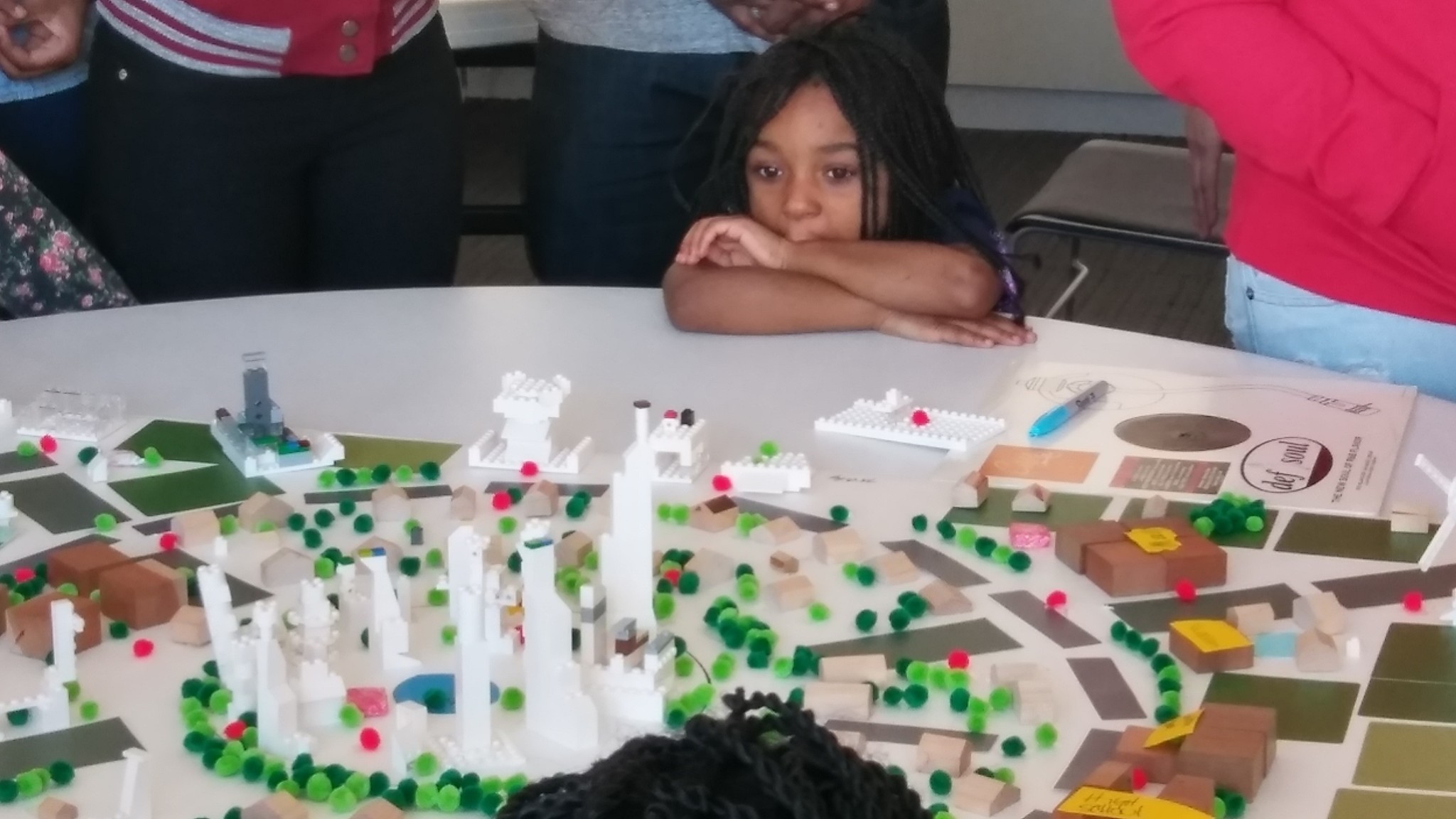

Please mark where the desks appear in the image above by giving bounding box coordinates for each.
[0,287,1456,819]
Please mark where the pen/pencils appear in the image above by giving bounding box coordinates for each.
[1027,379,1109,443]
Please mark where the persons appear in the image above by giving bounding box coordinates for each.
[1104,0,1456,404]
[0,0,471,323]
[660,5,1037,348]
[516,0,950,286]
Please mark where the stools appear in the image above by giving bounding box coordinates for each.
[1005,139,1238,319]
